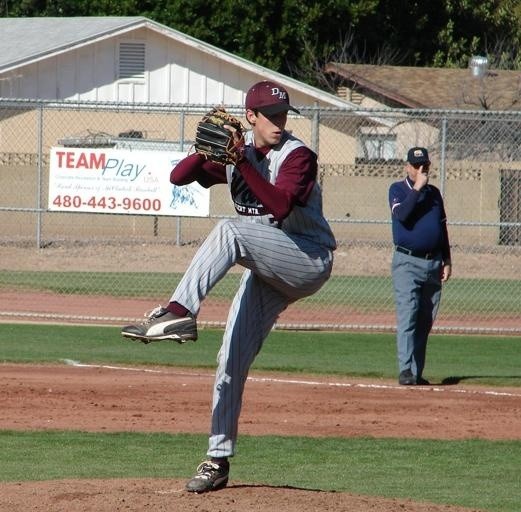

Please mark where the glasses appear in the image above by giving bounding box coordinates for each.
[413,164,429,169]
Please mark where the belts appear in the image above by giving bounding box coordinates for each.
[397,246,435,259]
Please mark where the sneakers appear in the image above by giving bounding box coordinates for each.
[186,460,230,493]
[121,304,197,344]
[399,370,429,385]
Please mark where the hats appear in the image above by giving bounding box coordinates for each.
[246,81,300,116]
[408,147,431,165]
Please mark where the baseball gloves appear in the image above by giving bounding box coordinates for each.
[194,103,245,166]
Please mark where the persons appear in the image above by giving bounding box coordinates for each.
[118,79,339,494]
[388,146,453,385]
